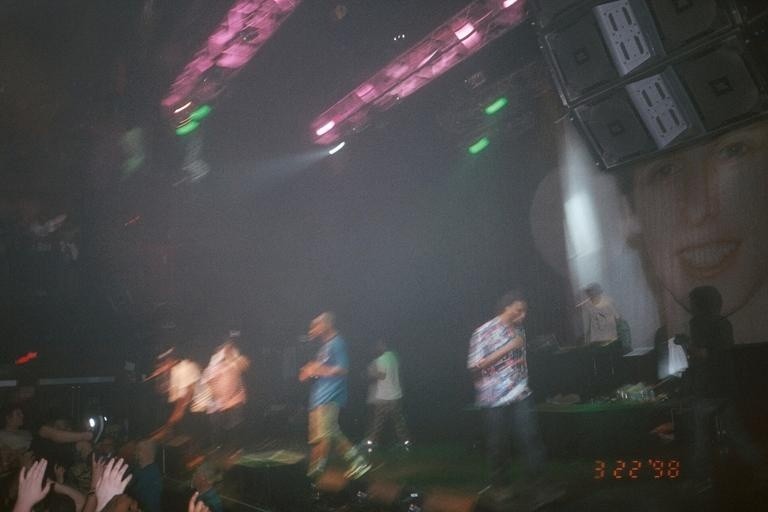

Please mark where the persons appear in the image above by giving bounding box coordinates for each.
[197,335,251,461]
[671,285,767,482]
[468,287,546,495]
[574,282,622,374]
[143,344,209,446]
[297,311,372,480]
[357,332,416,461]
[0,372,212,511]
[613,119,768,362]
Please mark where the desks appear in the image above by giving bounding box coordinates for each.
[536,403,665,421]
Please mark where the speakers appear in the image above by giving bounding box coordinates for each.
[543,29,768,170]
[542,0,768,107]
[222,448,308,511]
